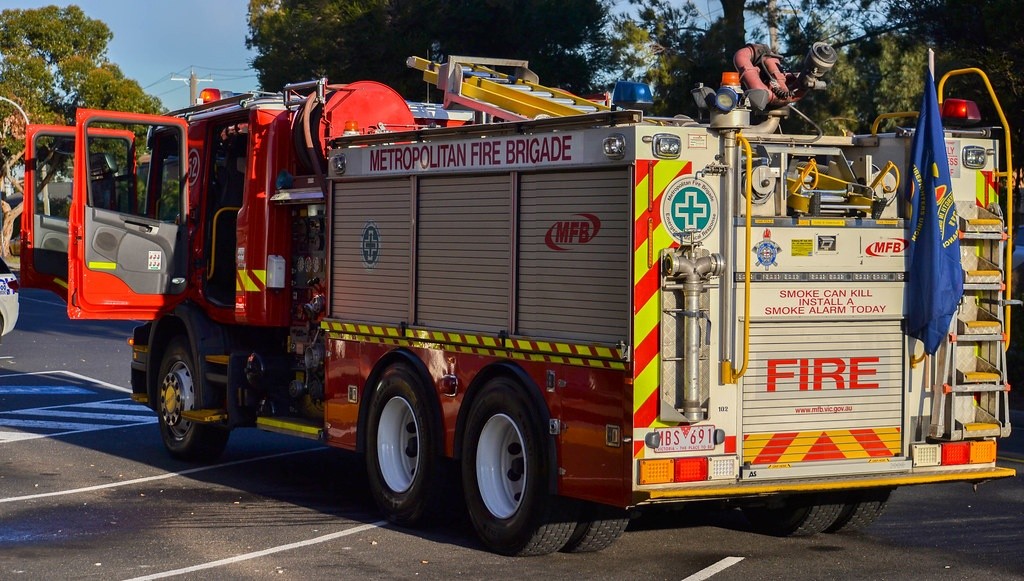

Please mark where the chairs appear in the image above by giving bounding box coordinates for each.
[211,132,248,269]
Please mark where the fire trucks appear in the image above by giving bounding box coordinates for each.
[17,40,1018,560]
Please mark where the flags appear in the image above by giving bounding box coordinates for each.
[907,66,963,356]
